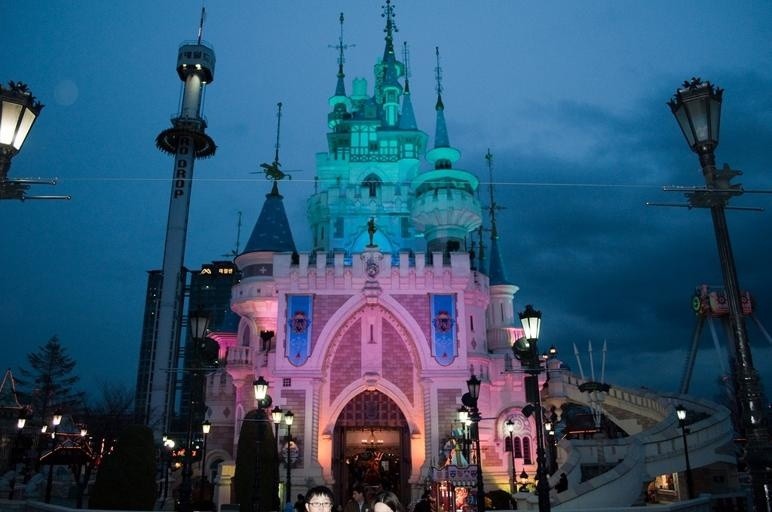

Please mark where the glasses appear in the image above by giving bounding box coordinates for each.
[309,503,332,508]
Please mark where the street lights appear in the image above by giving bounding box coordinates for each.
[249,374,295,512]
[5,407,212,512]
[674,402,693,471]
[2,76,76,207]
[454,300,557,512]
[667,69,771,512]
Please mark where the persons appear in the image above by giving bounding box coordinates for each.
[554,472,567,493]
[293,486,432,512]
